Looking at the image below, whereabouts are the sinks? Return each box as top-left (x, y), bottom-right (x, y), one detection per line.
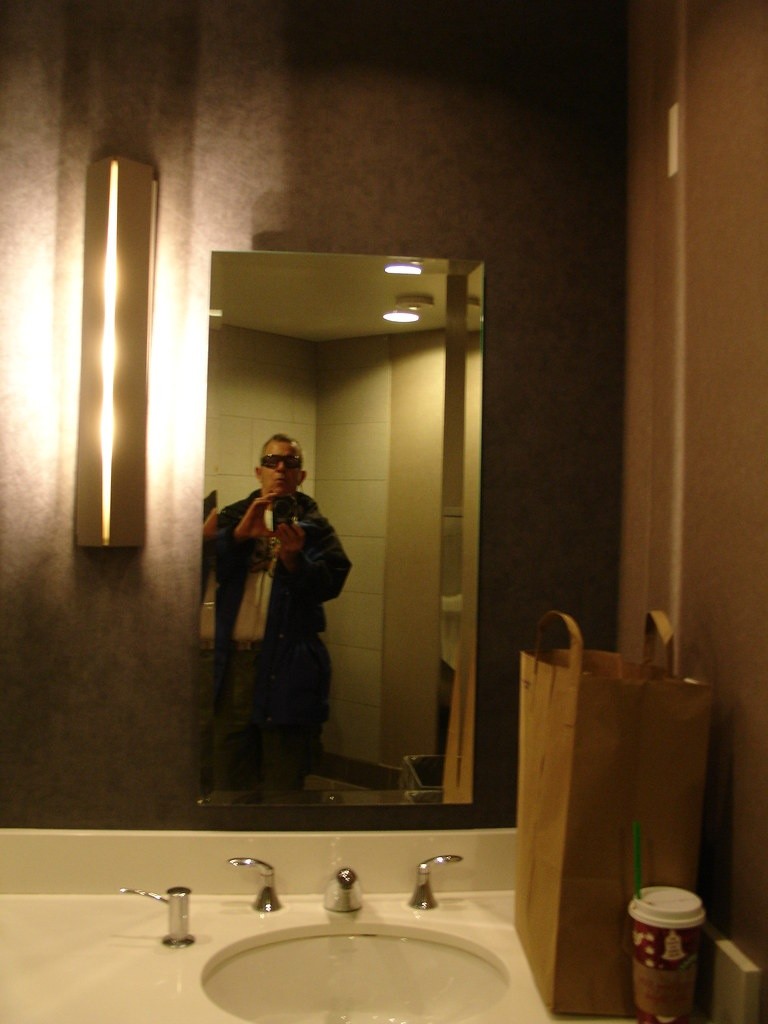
top-left (199, 920), bottom-right (510, 1024)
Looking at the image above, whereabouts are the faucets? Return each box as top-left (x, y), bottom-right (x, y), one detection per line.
top-left (323, 867), bottom-right (364, 911)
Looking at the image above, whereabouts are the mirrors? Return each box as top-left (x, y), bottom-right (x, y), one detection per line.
top-left (195, 249), bottom-right (484, 805)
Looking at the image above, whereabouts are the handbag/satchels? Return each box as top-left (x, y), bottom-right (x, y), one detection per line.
top-left (513, 609), bottom-right (711, 1017)
top-left (260, 634), bottom-right (331, 726)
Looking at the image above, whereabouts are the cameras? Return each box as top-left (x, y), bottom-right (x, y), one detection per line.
top-left (272, 495), bottom-right (297, 532)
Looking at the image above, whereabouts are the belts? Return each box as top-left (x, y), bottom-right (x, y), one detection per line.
top-left (233, 640), bottom-right (260, 651)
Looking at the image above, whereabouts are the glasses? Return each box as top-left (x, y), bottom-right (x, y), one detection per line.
top-left (262, 454), bottom-right (303, 468)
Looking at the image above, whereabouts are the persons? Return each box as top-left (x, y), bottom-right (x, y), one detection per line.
top-left (216, 433), bottom-right (353, 789)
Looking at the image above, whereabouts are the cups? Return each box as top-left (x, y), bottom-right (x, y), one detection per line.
top-left (630, 886), bottom-right (705, 1023)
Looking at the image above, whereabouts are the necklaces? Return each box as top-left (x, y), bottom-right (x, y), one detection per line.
top-left (269, 537), bottom-right (280, 576)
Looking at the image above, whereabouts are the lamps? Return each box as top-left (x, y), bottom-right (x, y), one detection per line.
top-left (72, 156), bottom-right (161, 550)
